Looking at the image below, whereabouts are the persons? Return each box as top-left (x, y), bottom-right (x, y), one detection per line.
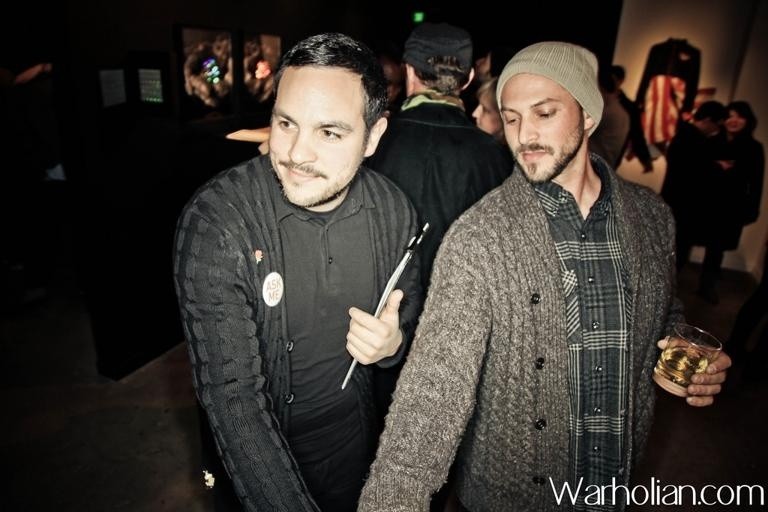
top-left (348, 38), bottom-right (736, 512)
top-left (170, 31), bottom-right (427, 512)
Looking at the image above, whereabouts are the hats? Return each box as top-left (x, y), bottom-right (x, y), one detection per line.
top-left (401, 21), bottom-right (472, 77)
top-left (496, 41), bottom-right (604, 137)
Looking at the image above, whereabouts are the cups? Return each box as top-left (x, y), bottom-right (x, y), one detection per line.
top-left (650, 323), bottom-right (723, 397)
